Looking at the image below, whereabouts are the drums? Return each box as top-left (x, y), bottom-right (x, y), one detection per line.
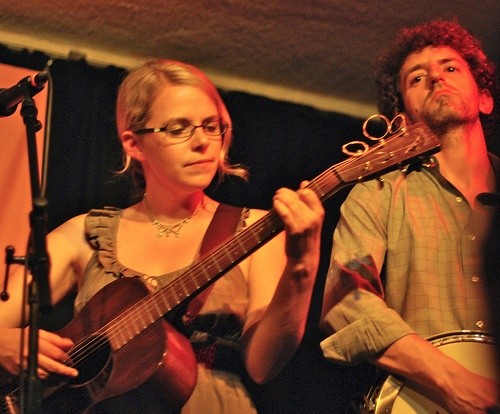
top-left (362, 330), bottom-right (500, 414)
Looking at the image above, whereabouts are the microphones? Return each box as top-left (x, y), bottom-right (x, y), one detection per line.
top-left (0, 72), bottom-right (48, 117)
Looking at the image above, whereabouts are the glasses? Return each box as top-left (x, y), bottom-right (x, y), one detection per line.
top-left (132, 118), bottom-right (230, 140)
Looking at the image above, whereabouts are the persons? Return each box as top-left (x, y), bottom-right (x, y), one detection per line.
top-left (318, 17), bottom-right (500, 414)
top-left (0, 58), bottom-right (326, 414)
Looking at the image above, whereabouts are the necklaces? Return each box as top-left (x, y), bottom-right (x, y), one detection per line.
top-left (143, 191), bottom-right (206, 238)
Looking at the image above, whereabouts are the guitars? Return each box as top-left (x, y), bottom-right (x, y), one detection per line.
top-left (0, 114), bottom-right (443, 414)
